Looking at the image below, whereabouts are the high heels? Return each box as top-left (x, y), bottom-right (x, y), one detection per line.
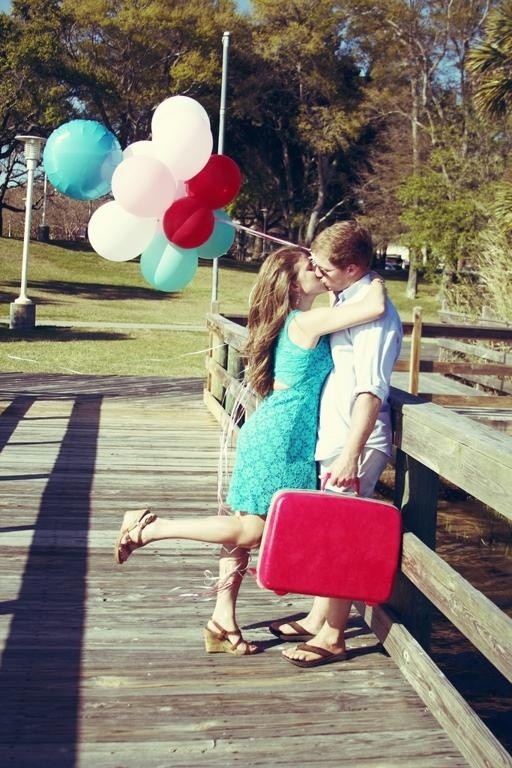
top-left (115, 509), bottom-right (158, 564)
top-left (203, 617), bottom-right (257, 655)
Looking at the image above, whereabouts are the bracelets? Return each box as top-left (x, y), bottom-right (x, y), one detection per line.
top-left (369, 278), bottom-right (385, 286)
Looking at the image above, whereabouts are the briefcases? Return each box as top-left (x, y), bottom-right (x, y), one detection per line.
top-left (256, 472), bottom-right (401, 606)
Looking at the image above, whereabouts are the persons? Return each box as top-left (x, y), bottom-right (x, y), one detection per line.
top-left (110, 245), bottom-right (389, 655)
top-left (264, 220), bottom-right (403, 667)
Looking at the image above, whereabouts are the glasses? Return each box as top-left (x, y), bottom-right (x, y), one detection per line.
top-left (316, 265), bottom-right (335, 276)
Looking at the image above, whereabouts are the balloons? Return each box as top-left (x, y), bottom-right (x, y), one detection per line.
top-left (45, 95), bottom-right (243, 292)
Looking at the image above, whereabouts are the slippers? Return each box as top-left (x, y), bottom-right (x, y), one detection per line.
top-left (269, 620), bottom-right (348, 668)
